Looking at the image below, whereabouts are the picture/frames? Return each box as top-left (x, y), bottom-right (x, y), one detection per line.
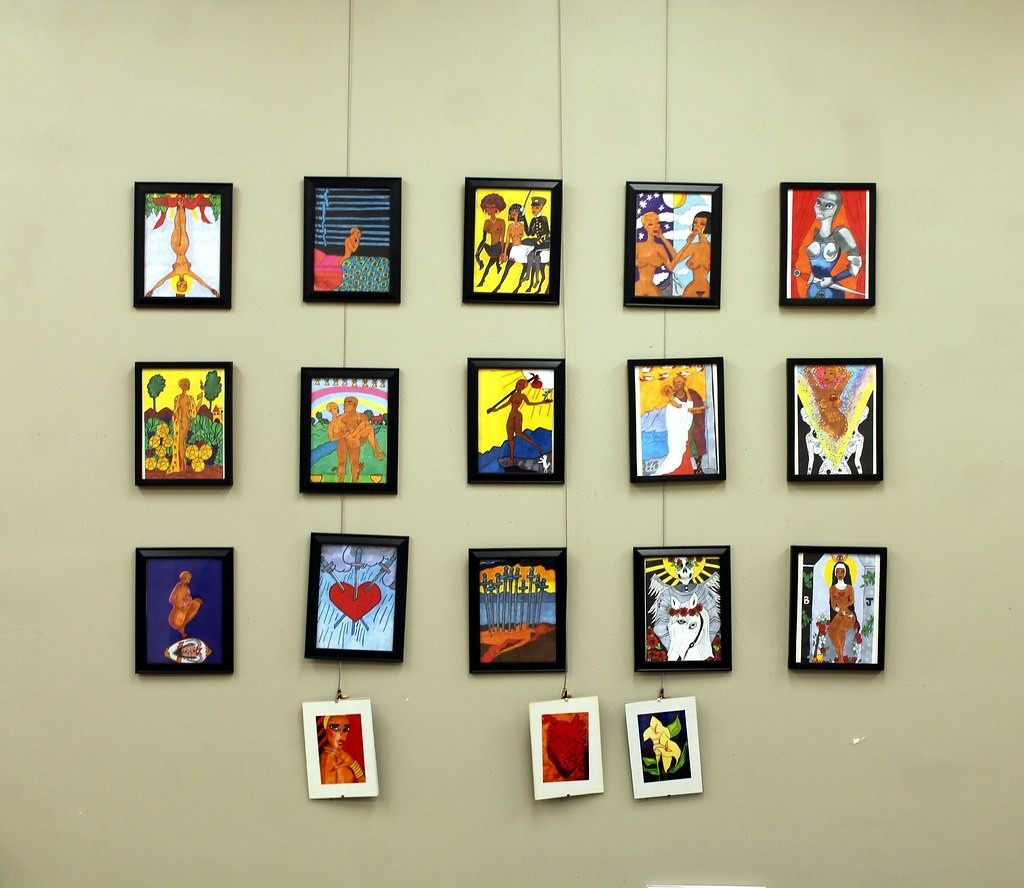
top-left (788, 544), bottom-right (887, 670)
top-left (134, 548), bottom-right (234, 675)
top-left (623, 180), bottom-right (722, 309)
top-left (467, 358), bottom-right (565, 484)
top-left (469, 547), bottom-right (568, 673)
top-left (134, 181), bottom-right (234, 309)
top-left (786, 358), bottom-right (883, 482)
top-left (305, 532), bottom-right (409, 663)
top-left (627, 357), bottom-right (726, 484)
top-left (463, 176), bottom-right (562, 305)
top-left (632, 545), bottom-right (733, 672)
top-left (779, 182), bottom-right (876, 307)
top-left (134, 362), bottom-right (234, 486)
top-left (303, 176), bottom-right (401, 304)
top-left (300, 367), bottom-right (399, 494)
